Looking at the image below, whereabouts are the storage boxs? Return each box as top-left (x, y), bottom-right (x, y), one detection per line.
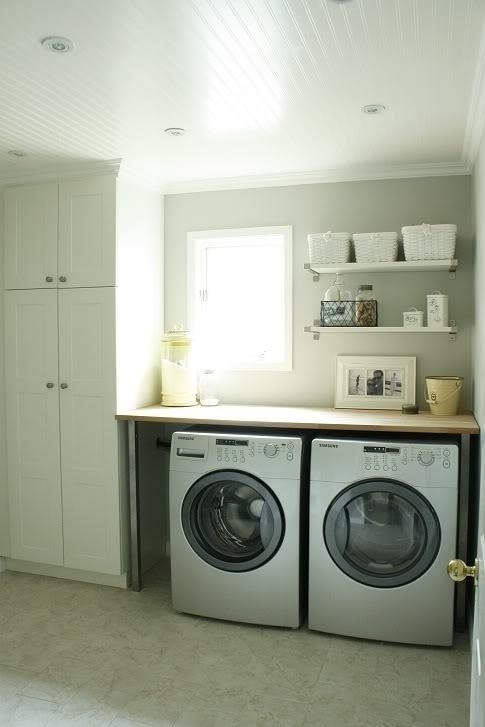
top-left (319, 300), bottom-right (378, 327)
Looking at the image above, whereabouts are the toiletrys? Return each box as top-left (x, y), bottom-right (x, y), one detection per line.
top-left (323, 273), bottom-right (353, 327)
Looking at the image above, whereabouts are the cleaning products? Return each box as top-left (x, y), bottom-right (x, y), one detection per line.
top-left (324, 271), bottom-right (356, 325)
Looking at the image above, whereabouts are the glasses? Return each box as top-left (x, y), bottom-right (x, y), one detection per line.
top-left (376, 376), bottom-right (381, 379)
top-left (367, 382), bottom-right (375, 387)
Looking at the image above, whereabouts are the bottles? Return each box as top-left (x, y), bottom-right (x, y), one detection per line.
top-left (355, 284), bottom-right (375, 326)
top-left (160, 322), bottom-right (198, 407)
top-left (198, 369), bottom-right (219, 406)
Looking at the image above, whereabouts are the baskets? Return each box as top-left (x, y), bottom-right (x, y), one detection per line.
top-left (308, 232), bottom-right (350, 264)
top-left (352, 231), bottom-right (398, 263)
top-left (401, 223), bottom-right (457, 261)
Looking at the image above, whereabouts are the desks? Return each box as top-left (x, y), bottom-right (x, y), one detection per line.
top-left (116, 399), bottom-right (479, 636)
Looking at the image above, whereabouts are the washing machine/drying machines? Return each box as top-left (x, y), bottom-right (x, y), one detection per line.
top-left (305, 438), bottom-right (459, 648)
top-left (167, 431), bottom-right (302, 632)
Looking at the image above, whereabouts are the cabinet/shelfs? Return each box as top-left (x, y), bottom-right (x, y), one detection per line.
top-left (0, 290), bottom-right (164, 592)
top-left (1, 159), bottom-right (166, 289)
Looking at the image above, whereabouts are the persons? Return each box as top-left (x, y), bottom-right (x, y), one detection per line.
top-left (389, 372), bottom-right (398, 396)
top-left (367, 370), bottom-right (383, 395)
top-left (356, 374), bottom-right (360, 389)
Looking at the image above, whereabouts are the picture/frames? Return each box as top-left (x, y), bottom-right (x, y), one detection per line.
top-left (335, 356), bottom-right (416, 412)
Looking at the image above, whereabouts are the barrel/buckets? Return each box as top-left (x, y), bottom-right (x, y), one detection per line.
top-left (423, 376), bottom-right (465, 416)
